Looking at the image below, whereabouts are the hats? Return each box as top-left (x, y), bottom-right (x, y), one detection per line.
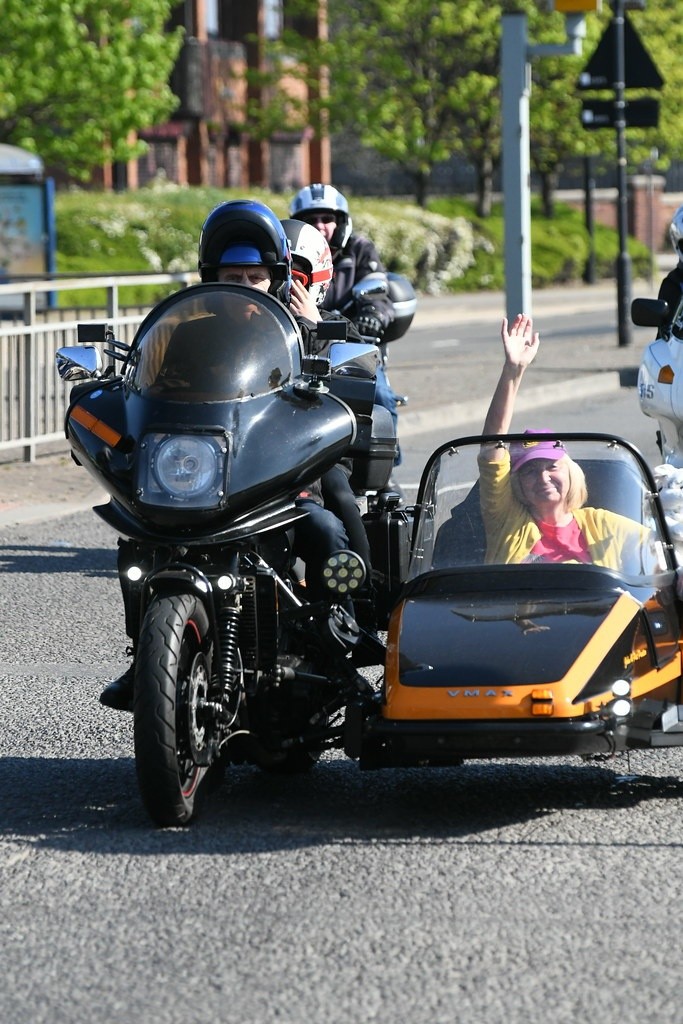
top-left (509, 430), bottom-right (565, 471)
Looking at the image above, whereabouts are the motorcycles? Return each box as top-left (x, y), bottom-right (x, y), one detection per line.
top-left (631, 284), bottom-right (682, 470)
top-left (52, 280), bottom-right (681, 828)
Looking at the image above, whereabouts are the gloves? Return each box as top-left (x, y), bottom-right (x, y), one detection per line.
top-left (355, 302), bottom-right (396, 339)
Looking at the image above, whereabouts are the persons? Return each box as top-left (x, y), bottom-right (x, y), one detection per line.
top-left (99, 200), bottom-right (372, 710)
top-left (277, 218), bottom-right (381, 668)
top-left (478, 314), bottom-right (667, 577)
top-left (654, 203), bottom-right (683, 343)
top-left (287, 184), bottom-right (405, 512)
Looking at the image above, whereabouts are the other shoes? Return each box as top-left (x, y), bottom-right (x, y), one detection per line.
top-left (100, 661), bottom-right (135, 712)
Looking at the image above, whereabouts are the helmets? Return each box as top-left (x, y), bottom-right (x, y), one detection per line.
top-left (669, 207), bottom-right (683, 262)
top-left (278, 218), bottom-right (333, 307)
top-left (198, 199), bottom-right (292, 308)
top-left (291, 183), bottom-right (354, 248)
